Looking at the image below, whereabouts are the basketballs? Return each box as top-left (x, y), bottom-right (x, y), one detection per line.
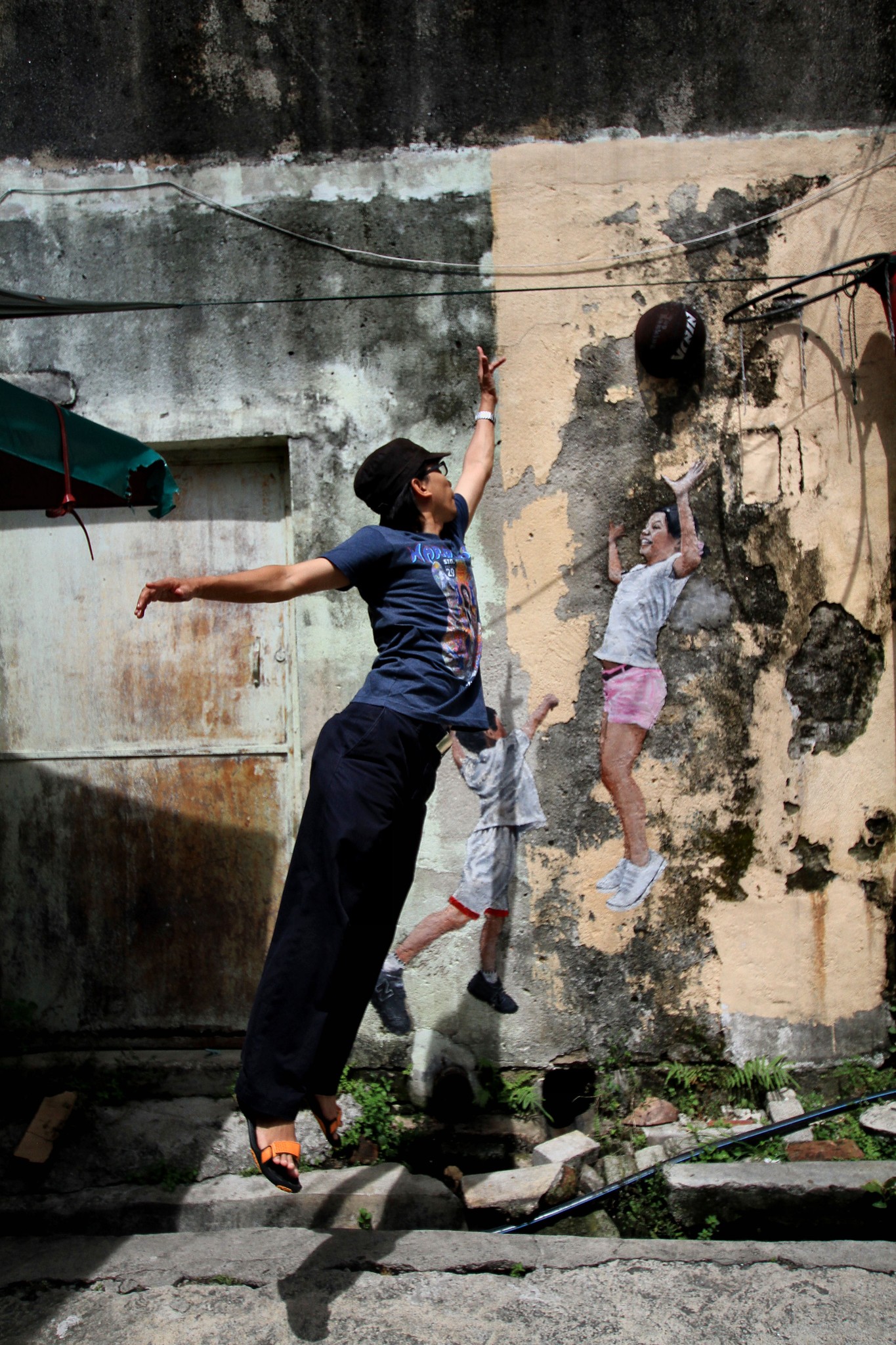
top-left (635, 301), bottom-right (705, 373)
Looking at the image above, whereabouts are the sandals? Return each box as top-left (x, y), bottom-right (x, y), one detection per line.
top-left (232, 1091), bottom-right (303, 1194)
top-left (307, 1093), bottom-right (342, 1149)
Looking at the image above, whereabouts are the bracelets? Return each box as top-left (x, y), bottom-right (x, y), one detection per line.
top-left (473, 410), bottom-right (497, 426)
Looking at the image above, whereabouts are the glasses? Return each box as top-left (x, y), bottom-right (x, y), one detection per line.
top-left (419, 461), bottom-right (448, 477)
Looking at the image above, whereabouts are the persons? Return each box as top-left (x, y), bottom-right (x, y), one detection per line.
top-left (131, 343), bottom-right (510, 1200)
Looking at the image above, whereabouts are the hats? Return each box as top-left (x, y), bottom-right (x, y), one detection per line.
top-left (353, 438), bottom-right (452, 516)
